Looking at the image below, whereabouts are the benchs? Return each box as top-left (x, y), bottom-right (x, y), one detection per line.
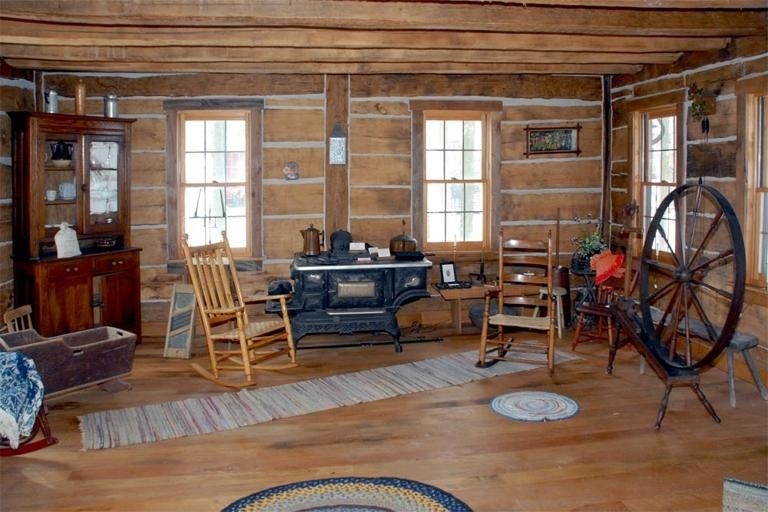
top-left (635, 299), bottom-right (767, 407)
top-left (608, 301), bottom-right (721, 430)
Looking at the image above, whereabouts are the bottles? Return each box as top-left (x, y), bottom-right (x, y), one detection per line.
top-left (106, 197), bottom-right (112, 223)
top-left (45, 88), bottom-right (57, 113)
top-left (74, 80), bottom-right (87, 115)
top-left (103, 85), bottom-right (120, 118)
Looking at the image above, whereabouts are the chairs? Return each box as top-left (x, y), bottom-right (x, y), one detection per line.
top-left (180, 232), bottom-right (298, 387)
top-left (0, 353), bottom-right (58, 458)
top-left (571, 266), bottom-right (639, 354)
top-left (2, 304), bottom-right (34, 330)
top-left (475, 227), bottom-right (561, 375)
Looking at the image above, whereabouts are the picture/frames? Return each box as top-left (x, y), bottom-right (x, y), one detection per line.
top-left (523, 124), bottom-right (582, 158)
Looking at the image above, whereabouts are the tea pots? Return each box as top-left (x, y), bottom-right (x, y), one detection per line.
top-left (300, 223), bottom-right (324, 256)
top-left (59, 176), bottom-right (76, 201)
top-left (51, 139), bottom-right (74, 160)
top-left (390, 218), bottom-right (417, 255)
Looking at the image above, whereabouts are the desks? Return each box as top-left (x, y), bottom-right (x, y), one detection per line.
top-left (570, 268), bottom-right (597, 329)
top-left (432, 282), bottom-right (491, 333)
top-left (267, 258), bottom-right (433, 355)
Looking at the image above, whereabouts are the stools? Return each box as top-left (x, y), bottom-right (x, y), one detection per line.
top-left (530, 286), bottom-right (566, 338)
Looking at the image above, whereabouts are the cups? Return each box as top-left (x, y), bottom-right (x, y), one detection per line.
top-left (46, 190), bottom-right (56, 201)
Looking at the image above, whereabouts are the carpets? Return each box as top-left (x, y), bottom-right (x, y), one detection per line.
top-left (78, 336), bottom-right (577, 452)
top-left (489, 390), bottom-right (580, 422)
top-left (218, 476), bottom-right (474, 512)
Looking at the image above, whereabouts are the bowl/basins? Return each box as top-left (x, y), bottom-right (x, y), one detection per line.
top-left (53, 160), bottom-right (71, 167)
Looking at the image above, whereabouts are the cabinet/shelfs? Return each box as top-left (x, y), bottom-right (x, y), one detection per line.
top-left (6, 110), bottom-right (141, 346)
